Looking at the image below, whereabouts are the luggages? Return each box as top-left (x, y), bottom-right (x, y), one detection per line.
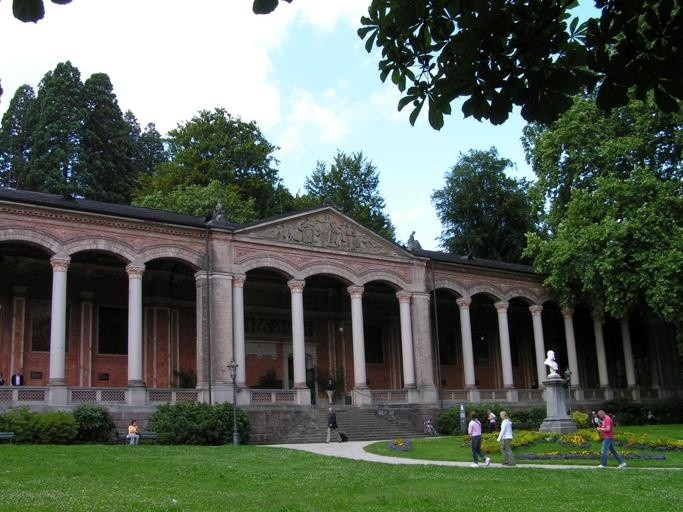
top-left (338, 432), bottom-right (348, 443)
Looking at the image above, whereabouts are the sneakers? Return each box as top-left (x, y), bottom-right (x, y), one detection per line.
top-left (485, 457), bottom-right (491, 467)
top-left (617, 463), bottom-right (626, 469)
top-left (503, 461), bottom-right (516, 466)
top-left (597, 464), bottom-right (607, 468)
top-left (470, 464), bottom-right (479, 468)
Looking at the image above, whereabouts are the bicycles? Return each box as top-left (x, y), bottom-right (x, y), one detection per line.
top-left (422, 415), bottom-right (438, 437)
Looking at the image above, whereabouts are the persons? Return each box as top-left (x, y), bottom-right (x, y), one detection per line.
top-left (10, 370), bottom-right (23, 385)
top-left (543, 349), bottom-right (558, 371)
top-left (596, 409), bottom-right (627, 468)
top-left (495, 411), bottom-right (514, 466)
top-left (467, 410), bottom-right (490, 468)
top-left (126, 420), bottom-right (139, 446)
top-left (487, 409), bottom-right (498, 433)
top-left (324, 377), bottom-right (335, 404)
top-left (325, 407), bottom-right (342, 442)
top-left (0, 372), bottom-right (3, 385)
top-left (591, 410), bottom-right (616, 427)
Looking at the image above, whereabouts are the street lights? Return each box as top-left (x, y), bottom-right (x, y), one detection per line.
top-left (225, 357), bottom-right (242, 445)
top-left (563, 365), bottom-right (573, 416)
